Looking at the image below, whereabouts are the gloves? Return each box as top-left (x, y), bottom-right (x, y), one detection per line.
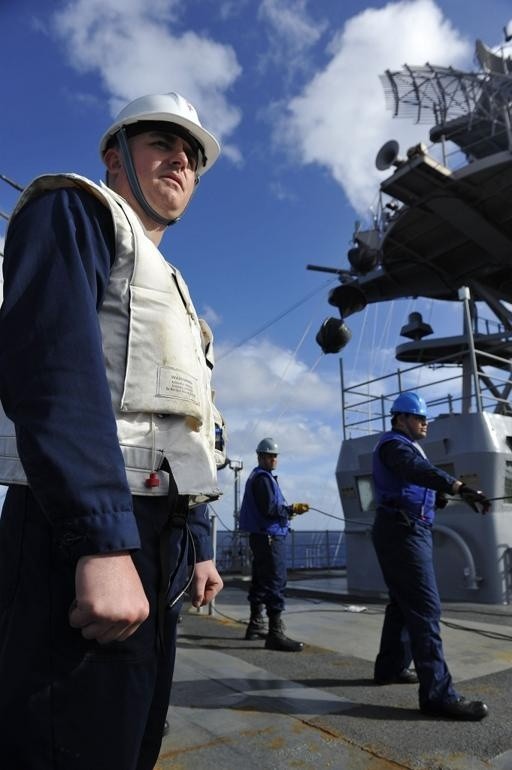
top-left (458, 483), bottom-right (491, 515)
top-left (292, 503), bottom-right (310, 515)
top-left (436, 491), bottom-right (447, 509)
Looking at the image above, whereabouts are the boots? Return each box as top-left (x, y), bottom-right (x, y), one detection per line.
top-left (245, 602), bottom-right (270, 641)
top-left (265, 611), bottom-right (304, 652)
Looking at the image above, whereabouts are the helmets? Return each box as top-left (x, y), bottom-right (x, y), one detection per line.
top-left (255, 438), bottom-right (280, 454)
top-left (390, 391), bottom-right (427, 417)
top-left (99, 92), bottom-right (221, 183)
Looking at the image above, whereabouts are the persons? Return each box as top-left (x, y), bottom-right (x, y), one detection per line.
top-left (236, 437), bottom-right (311, 653)
top-left (0, 87), bottom-right (227, 770)
top-left (369, 390), bottom-right (492, 723)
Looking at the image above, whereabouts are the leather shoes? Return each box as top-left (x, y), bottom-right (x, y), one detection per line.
top-left (373, 668), bottom-right (419, 685)
top-left (422, 695), bottom-right (487, 721)
